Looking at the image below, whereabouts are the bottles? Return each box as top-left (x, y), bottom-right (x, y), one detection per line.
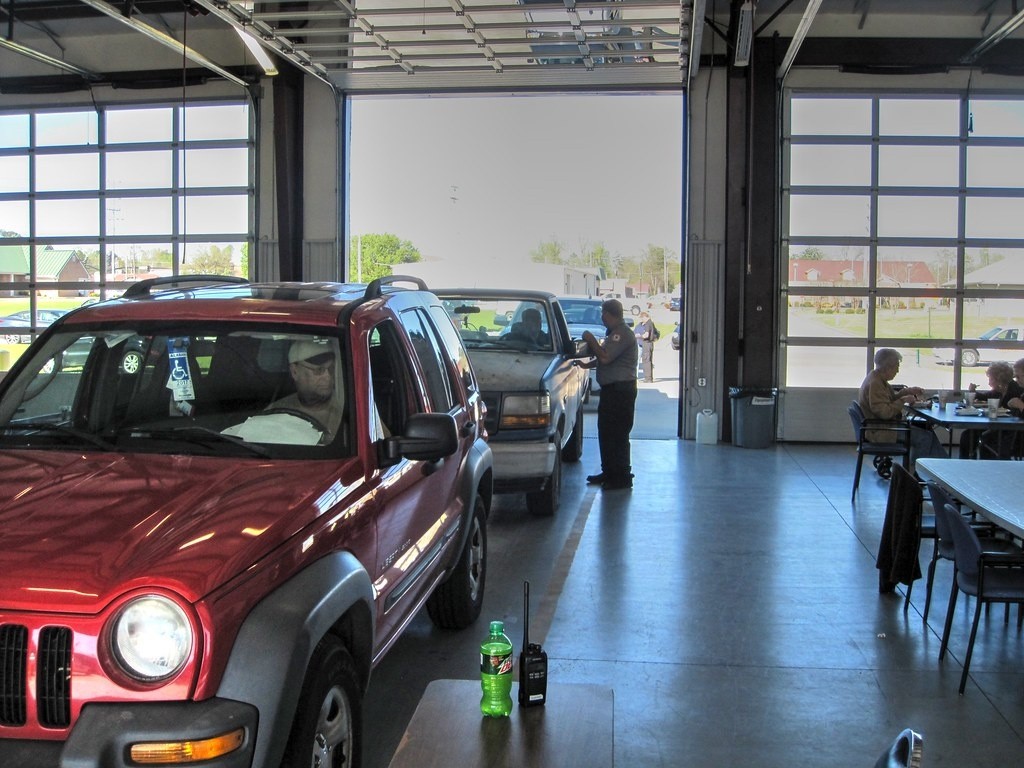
top-left (480, 621), bottom-right (513, 718)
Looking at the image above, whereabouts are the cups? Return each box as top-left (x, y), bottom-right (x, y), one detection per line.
top-left (988, 399), bottom-right (1000, 418)
top-left (965, 393), bottom-right (976, 408)
top-left (938, 391), bottom-right (949, 408)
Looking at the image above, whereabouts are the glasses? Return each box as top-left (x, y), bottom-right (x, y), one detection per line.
top-left (295, 363), bottom-right (335, 376)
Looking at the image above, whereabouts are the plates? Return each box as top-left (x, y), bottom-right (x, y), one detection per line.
top-left (973, 401), bottom-right (988, 408)
top-left (909, 402), bottom-right (933, 408)
top-left (986, 408), bottom-right (1011, 414)
top-left (953, 409), bottom-right (983, 415)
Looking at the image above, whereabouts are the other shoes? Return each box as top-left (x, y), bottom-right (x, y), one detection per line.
top-left (586, 470), bottom-right (606, 483)
top-left (601, 471), bottom-right (633, 490)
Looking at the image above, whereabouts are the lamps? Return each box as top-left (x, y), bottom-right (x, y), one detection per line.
top-left (233, 25), bottom-right (278, 75)
top-left (733, 0), bottom-right (756, 67)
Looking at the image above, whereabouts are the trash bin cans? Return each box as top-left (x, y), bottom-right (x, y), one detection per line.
top-left (729, 386), bottom-right (777, 449)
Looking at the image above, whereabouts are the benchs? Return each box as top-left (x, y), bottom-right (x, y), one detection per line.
top-left (180, 338), bottom-right (397, 419)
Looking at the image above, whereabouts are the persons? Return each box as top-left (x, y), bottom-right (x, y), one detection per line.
top-left (576, 299), bottom-right (638, 490)
top-left (507, 308), bottom-right (551, 347)
top-left (587, 309), bottom-right (602, 324)
top-left (859, 349), bottom-right (948, 485)
top-left (265, 340), bottom-right (390, 442)
top-left (633, 312), bottom-right (654, 383)
top-left (959, 358), bottom-right (1024, 461)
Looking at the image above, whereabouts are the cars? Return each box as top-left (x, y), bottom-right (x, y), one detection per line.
top-left (0, 309), bottom-right (162, 377)
top-left (672, 321), bottom-right (681, 350)
top-left (494, 297), bottom-right (634, 396)
top-left (669, 298), bottom-right (681, 311)
top-left (933, 324), bottom-right (1024, 368)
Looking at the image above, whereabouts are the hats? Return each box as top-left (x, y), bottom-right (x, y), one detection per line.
top-left (287, 339), bottom-right (336, 364)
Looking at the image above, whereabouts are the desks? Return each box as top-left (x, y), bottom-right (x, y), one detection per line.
top-left (389, 679), bottom-right (615, 768)
top-left (916, 457), bottom-right (1024, 624)
top-left (903, 401), bottom-right (1024, 527)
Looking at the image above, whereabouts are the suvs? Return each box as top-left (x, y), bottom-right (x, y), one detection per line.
top-left (0, 274), bottom-right (496, 768)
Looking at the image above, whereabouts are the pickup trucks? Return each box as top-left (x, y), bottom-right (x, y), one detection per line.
top-left (428, 288), bottom-right (591, 519)
top-left (598, 290), bottom-right (649, 317)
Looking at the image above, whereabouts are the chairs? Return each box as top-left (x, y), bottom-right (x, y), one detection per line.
top-left (111, 353), bottom-right (208, 430)
top-left (847, 400), bottom-right (1024, 695)
top-left (510, 320), bottom-right (524, 335)
top-left (275, 350), bottom-right (393, 430)
